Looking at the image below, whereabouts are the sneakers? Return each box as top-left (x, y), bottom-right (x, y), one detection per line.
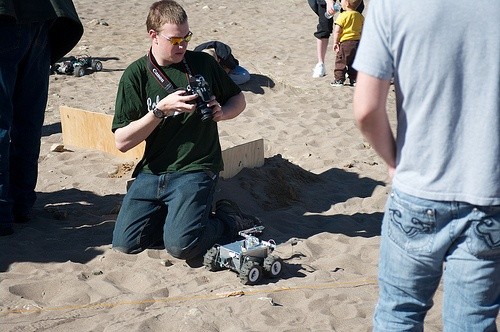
top-left (215, 198), bottom-right (260, 230)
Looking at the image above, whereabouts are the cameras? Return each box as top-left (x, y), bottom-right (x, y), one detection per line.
top-left (185, 78), bottom-right (214, 122)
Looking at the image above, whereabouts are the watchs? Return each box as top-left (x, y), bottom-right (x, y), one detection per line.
top-left (152, 106), bottom-right (166, 123)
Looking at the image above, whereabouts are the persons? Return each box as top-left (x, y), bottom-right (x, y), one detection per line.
top-left (111, 0), bottom-right (263, 261)
top-left (308, 0), bottom-right (365, 78)
top-left (331, 0), bottom-right (365, 88)
top-left (352, 0), bottom-right (500, 332)
top-left (0, 0), bottom-right (83, 232)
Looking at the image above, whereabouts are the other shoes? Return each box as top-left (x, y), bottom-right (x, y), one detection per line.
top-left (331, 80), bottom-right (343, 87)
top-left (313, 63), bottom-right (326, 79)
top-left (0, 205), bottom-right (15, 235)
top-left (13, 191), bottom-right (37, 224)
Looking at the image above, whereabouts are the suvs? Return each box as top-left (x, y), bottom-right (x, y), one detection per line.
top-left (202, 225), bottom-right (284, 286)
top-left (49, 56), bottom-right (102, 77)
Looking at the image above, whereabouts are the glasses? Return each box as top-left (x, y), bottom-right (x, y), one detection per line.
top-left (158, 30), bottom-right (193, 46)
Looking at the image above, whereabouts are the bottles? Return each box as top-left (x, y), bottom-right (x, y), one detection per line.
top-left (324, 3), bottom-right (341, 19)
